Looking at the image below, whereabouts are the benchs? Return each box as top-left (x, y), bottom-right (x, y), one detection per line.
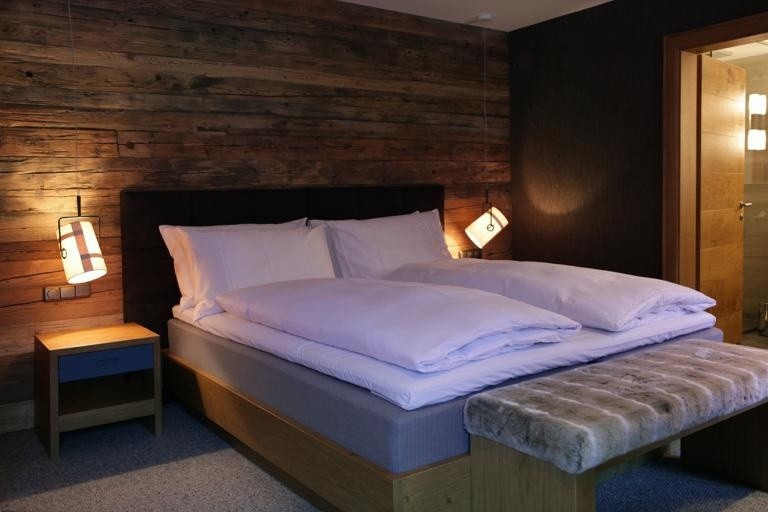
top-left (462, 336), bottom-right (768, 512)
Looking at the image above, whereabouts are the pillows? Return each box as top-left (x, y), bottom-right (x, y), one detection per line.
top-left (155, 216), bottom-right (309, 314)
top-left (304, 211), bottom-right (421, 279)
top-left (321, 209), bottom-right (454, 281)
top-left (169, 223), bottom-right (340, 328)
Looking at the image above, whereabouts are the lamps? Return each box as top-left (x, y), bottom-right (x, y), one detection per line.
top-left (462, 9), bottom-right (511, 253)
top-left (50, 1), bottom-right (108, 289)
top-left (745, 91), bottom-right (768, 151)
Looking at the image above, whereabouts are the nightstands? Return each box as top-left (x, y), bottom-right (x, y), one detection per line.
top-left (34, 321), bottom-right (164, 463)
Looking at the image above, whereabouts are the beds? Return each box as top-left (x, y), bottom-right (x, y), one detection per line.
top-left (113, 178), bottom-right (725, 512)
